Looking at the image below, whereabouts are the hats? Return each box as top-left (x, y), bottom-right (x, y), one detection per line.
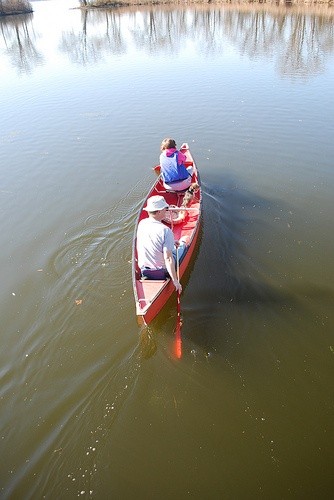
top-left (143, 195), bottom-right (169, 211)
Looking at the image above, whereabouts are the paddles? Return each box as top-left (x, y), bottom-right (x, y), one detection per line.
top-left (174, 242), bottom-right (182, 360)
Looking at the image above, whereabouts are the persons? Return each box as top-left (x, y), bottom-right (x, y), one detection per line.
top-left (160, 138), bottom-right (193, 191)
top-left (136, 195), bottom-right (189, 295)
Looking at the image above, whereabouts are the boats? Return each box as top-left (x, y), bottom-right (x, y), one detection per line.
top-left (131, 143), bottom-right (202, 330)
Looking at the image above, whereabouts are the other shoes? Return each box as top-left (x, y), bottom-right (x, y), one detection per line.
top-left (179, 236), bottom-right (191, 245)
